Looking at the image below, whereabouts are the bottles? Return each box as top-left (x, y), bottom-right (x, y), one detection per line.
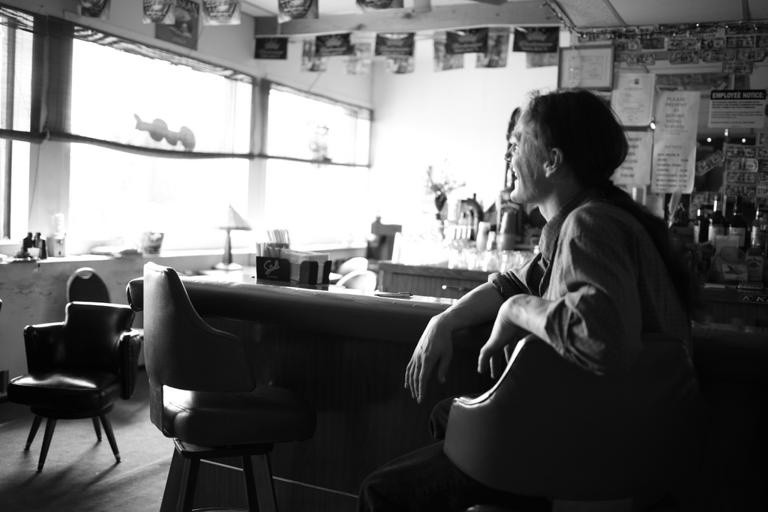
top-left (708, 195), bottom-right (725, 243)
top-left (750, 205), bottom-right (764, 252)
top-left (693, 208), bottom-right (707, 244)
top-left (729, 195), bottom-right (747, 251)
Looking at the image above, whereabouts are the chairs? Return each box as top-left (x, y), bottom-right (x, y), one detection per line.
top-left (442, 333), bottom-right (695, 512)
top-left (7, 300), bottom-right (143, 474)
top-left (66, 267), bottom-right (144, 339)
top-left (144, 260), bottom-right (316, 512)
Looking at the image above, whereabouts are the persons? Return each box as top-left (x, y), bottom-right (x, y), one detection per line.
top-left (355, 88), bottom-right (703, 512)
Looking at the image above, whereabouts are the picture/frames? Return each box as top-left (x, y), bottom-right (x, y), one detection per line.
top-left (557, 44), bottom-right (615, 92)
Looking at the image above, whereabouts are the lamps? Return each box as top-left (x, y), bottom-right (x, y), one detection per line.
top-left (211, 204), bottom-right (251, 270)
